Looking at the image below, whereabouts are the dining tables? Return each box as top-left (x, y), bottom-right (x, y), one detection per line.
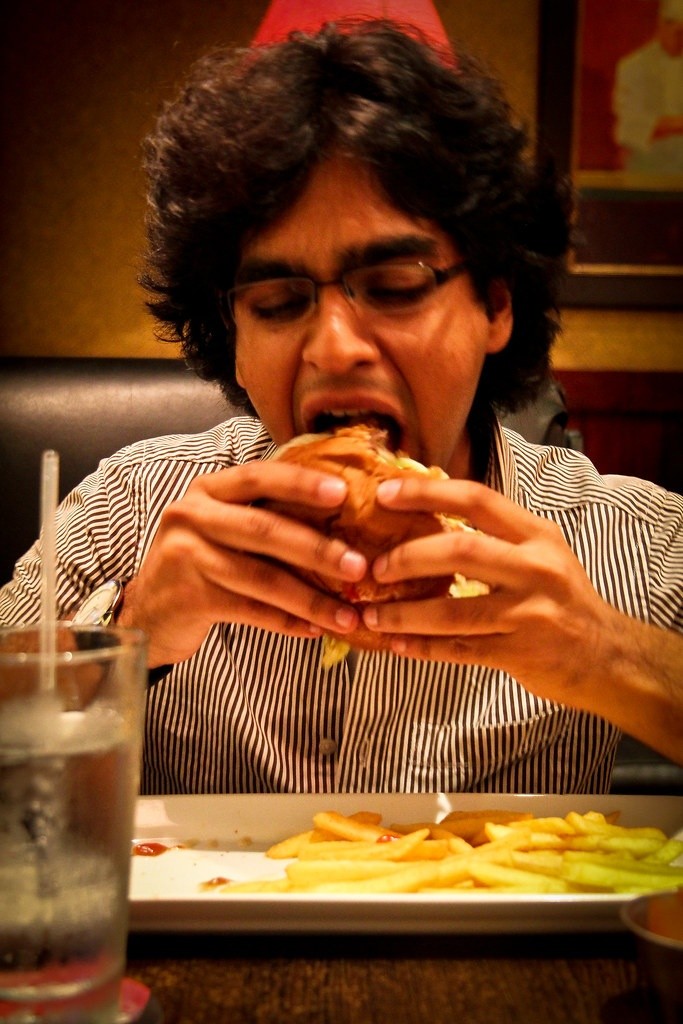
top-left (124, 930), bottom-right (683, 1024)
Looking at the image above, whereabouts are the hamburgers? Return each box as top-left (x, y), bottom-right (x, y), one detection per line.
top-left (261, 422), bottom-right (498, 671)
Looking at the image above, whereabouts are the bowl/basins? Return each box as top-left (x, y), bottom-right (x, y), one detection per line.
top-left (619, 889), bottom-right (682, 1010)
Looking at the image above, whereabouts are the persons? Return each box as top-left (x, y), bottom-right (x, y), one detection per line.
top-left (0, 14), bottom-right (683, 795)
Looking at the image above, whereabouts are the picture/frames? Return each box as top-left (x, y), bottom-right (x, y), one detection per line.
top-left (531, 0), bottom-right (683, 315)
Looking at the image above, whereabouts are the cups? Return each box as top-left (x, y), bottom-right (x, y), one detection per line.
top-left (1, 618), bottom-right (148, 1021)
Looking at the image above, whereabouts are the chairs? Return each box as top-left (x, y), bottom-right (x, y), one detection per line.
top-left (0, 356), bottom-right (248, 591)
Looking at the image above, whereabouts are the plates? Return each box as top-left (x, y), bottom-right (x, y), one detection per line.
top-left (103, 788), bottom-right (682, 936)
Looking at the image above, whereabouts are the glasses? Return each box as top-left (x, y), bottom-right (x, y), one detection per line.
top-left (222, 247), bottom-right (489, 334)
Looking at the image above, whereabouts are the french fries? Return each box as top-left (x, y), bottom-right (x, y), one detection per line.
top-left (222, 811), bottom-right (683, 896)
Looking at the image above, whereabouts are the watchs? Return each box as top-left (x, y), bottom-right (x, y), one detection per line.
top-left (67, 573), bottom-right (174, 693)
